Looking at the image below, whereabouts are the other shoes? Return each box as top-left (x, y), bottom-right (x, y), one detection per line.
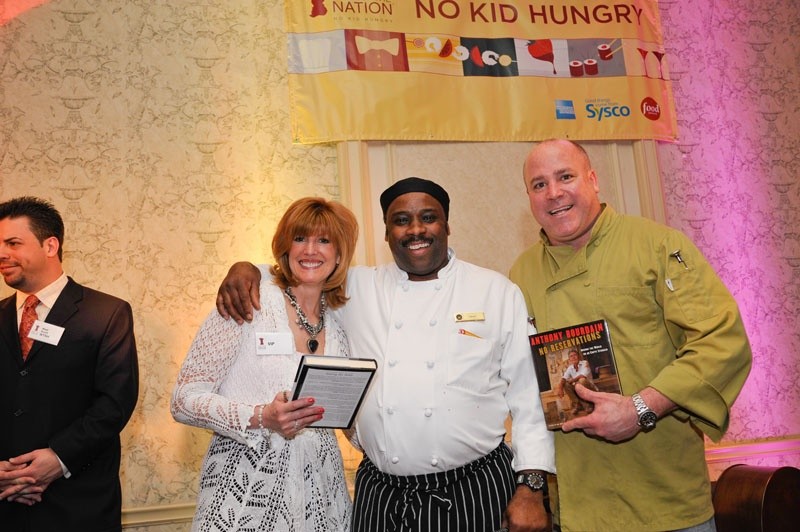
top-left (585, 402), bottom-right (595, 413)
top-left (572, 403), bottom-right (583, 415)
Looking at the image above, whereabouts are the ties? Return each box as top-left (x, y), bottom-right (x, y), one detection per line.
top-left (18, 293), bottom-right (39, 361)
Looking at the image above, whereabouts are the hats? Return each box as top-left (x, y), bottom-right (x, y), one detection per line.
top-left (380, 176), bottom-right (449, 220)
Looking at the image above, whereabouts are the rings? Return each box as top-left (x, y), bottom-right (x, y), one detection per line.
top-left (294, 420), bottom-right (302, 429)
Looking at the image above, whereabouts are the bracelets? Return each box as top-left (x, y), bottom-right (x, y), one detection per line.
top-left (257, 404), bottom-right (274, 450)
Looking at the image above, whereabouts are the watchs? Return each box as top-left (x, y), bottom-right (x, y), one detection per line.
top-left (515, 474), bottom-right (548, 491)
top-left (632, 393), bottom-right (658, 431)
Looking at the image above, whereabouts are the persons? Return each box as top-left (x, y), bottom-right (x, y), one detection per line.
top-left (1, 195), bottom-right (141, 532)
top-left (553, 348), bottom-right (599, 421)
top-left (172, 202), bottom-right (367, 532)
top-left (217, 176), bottom-right (560, 532)
top-left (501, 137), bottom-right (753, 532)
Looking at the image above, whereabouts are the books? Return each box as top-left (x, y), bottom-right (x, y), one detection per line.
top-left (288, 355), bottom-right (378, 430)
top-left (524, 320), bottom-right (624, 432)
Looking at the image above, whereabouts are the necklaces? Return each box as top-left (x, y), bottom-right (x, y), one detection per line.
top-left (284, 285), bottom-right (329, 354)
